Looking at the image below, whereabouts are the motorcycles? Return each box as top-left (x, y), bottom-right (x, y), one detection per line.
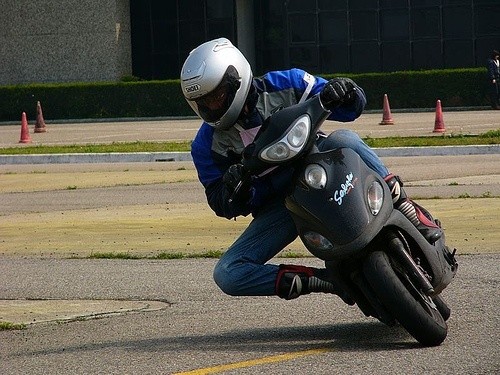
top-left (201, 83), bottom-right (458, 346)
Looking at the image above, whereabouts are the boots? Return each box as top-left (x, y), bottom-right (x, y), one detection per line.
top-left (383, 172), bottom-right (442, 245)
top-left (275, 264), bottom-right (355, 305)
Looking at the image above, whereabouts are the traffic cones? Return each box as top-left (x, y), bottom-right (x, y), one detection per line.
top-left (433, 100), bottom-right (446, 132)
top-left (33, 100), bottom-right (47, 133)
top-left (379, 94), bottom-right (394, 125)
top-left (18, 112), bottom-right (32, 143)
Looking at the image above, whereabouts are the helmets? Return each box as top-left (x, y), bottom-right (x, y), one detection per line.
top-left (180, 38), bottom-right (252, 130)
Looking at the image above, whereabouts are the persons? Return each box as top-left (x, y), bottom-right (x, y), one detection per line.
top-left (487, 50), bottom-right (500, 109)
top-left (180, 36), bottom-right (447, 307)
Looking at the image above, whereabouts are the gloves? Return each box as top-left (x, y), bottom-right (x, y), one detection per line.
top-left (324, 77), bottom-right (356, 106)
top-left (220, 165), bottom-right (255, 202)
